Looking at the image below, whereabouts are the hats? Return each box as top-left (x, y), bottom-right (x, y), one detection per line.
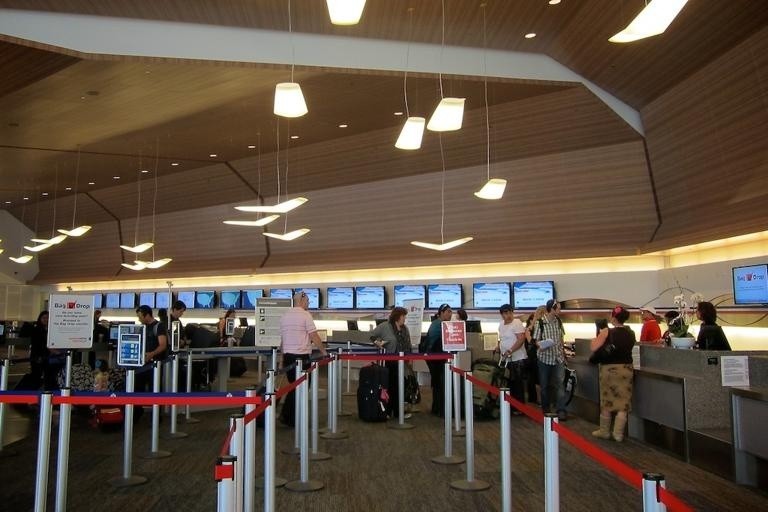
top-left (639, 307), bottom-right (656, 315)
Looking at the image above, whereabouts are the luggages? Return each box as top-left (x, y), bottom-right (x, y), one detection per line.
top-left (356, 346), bottom-right (392, 422)
top-left (472, 349), bottom-right (511, 416)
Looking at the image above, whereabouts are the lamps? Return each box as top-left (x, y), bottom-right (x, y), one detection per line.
top-left (410, 133), bottom-right (474, 251)
top-left (525, 33), bottom-right (536, 38)
top-left (210, 154), bottom-right (217, 157)
top-left (327, 1), bottom-right (365, 25)
top-left (222, 114), bottom-right (311, 240)
top-left (274, 1), bottom-right (308, 118)
top-left (340, 124), bottom-right (347, 127)
top-left (88, 182), bottom-right (95, 186)
top-left (394, 111), bottom-right (403, 115)
top-left (395, 8), bottom-right (425, 150)
top-left (8, 146), bottom-right (92, 264)
top-left (474, 4), bottom-right (507, 200)
top-left (114, 177), bottom-right (121, 179)
top-left (172, 163), bottom-right (179, 166)
top-left (426, 1), bottom-right (465, 133)
top-left (608, 1), bottom-right (688, 43)
top-left (549, 1), bottom-right (560, 5)
top-left (120, 138), bottom-right (172, 271)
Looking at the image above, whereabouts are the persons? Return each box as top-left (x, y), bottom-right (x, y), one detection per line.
top-left (661, 311), bottom-right (694, 348)
top-left (423, 303), bottom-right (452, 417)
top-left (279, 291), bottom-right (327, 426)
top-left (136, 301), bottom-right (191, 394)
top-left (217, 310), bottom-right (237, 347)
top-left (494, 299), bottom-right (568, 422)
top-left (369, 307), bottom-right (413, 420)
top-left (591, 306), bottom-right (636, 443)
top-left (30, 311), bottom-right (53, 386)
top-left (94, 310), bottom-right (104, 342)
top-left (639, 304), bottom-right (663, 343)
top-left (689, 303), bottom-right (731, 349)
top-left (88, 360), bottom-right (109, 410)
top-left (456, 308), bottom-right (468, 322)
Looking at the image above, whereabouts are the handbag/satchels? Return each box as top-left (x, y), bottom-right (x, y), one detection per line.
top-left (405, 364), bottom-right (421, 405)
top-left (588, 327), bottom-right (615, 365)
top-left (359, 364), bottom-right (390, 388)
top-left (564, 364), bottom-right (577, 392)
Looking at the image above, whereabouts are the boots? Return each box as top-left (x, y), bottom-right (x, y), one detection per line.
top-left (591, 414), bottom-right (612, 440)
top-left (610, 416), bottom-right (628, 442)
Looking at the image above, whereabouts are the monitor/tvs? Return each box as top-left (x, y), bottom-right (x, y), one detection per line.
top-left (427, 284), bottom-right (462, 309)
top-left (94, 289), bottom-right (293, 310)
top-left (355, 286), bottom-right (385, 309)
top-left (732, 263), bottom-right (768, 304)
top-left (472, 282), bottom-right (511, 309)
top-left (513, 281), bottom-right (554, 310)
top-left (326, 287), bottom-right (354, 309)
top-left (294, 288), bottom-right (320, 309)
top-left (394, 285), bottom-right (426, 309)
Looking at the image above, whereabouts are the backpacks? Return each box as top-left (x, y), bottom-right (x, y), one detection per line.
top-left (153, 321), bottom-right (172, 362)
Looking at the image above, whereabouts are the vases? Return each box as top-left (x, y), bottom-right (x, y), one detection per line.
top-left (669, 337), bottom-right (698, 351)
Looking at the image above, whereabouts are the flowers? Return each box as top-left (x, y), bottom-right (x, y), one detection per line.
top-left (662, 288), bottom-right (705, 337)
